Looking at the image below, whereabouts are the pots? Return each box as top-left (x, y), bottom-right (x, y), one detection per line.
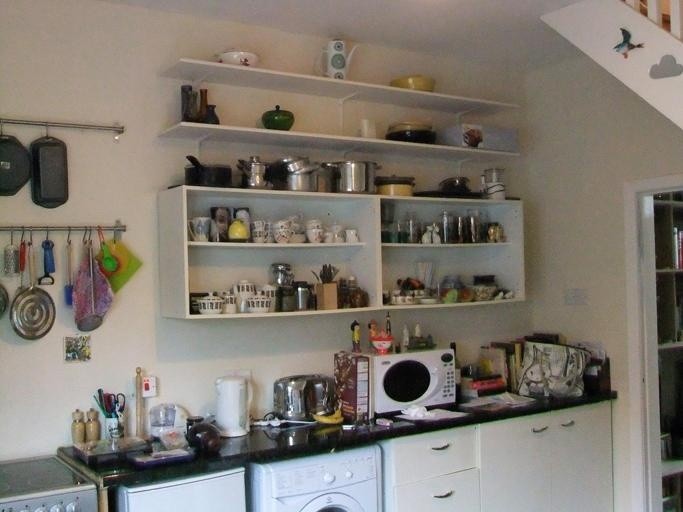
top-left (184, 154), bottom-right (381, 192)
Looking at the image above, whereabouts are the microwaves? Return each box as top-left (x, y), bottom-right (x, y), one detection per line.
top-left (373, 347), bottom-right (456, 414)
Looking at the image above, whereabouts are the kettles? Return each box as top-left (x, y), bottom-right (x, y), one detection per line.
top-left (213, 376), bottom-right (253, 438)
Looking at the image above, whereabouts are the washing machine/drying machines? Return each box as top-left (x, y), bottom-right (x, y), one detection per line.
top-left (245, 443), bottom-right (383, 512)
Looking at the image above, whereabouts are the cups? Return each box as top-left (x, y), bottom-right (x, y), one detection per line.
top-left (188, 211), bottom-right (360, 244)
top-left (106, 417), bottom-right (123, 440)
top-left (262, 285), bottom-right (276, 310)
top-left (220, 292), bottom-right (237, 313)
top-left (484, 167), bottom-right (507, 200)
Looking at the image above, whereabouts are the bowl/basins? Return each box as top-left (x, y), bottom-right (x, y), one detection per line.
top-left (213, 50), bottom-right (259, 66)
top-left (469, 285), bottom-right (497, 301)
top-left (246, 300), bottom-right (271, 314)
top-left (385, 122), bottom-right (436, 143)
top-left (389, 72), bottom-right (438, 91)
top-left (197, 300), bottom-right (223, 314)
top-left (370, 337), bottom-right (393, 354)
top-left (376, 176), bottom-right (415, 197)
top-left (184, 424), bottom-right (222, 456)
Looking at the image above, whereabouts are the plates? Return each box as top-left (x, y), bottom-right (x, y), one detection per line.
top-left (415, 297), bottom-right (440, 304)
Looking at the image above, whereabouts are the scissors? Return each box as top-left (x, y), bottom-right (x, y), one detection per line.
top-left (109, 393), bottom-right (125, 413)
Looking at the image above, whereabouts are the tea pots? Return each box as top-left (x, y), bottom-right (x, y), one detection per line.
top-left (232, 279), bottom-right (255, 312)
top-left (317, 37), bottom-right (357, 81)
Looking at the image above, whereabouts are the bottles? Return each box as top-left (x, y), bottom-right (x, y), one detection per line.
top-left (461, 345), bottom-right (492, 400)
top-left (271, 261), bottom-right (310, 310)
top-left (401, 210), bottom-right (419, 242)
top-left (187, 416), bottom-right (203, 433)
top-left (439, 210), bottom-right (482, 244)
top-left (182, 85), bottom-right (218, 124)
top-left (71, 409), bottom-right (101, 442)
top-left (441, 282), bottom-right (454, 304)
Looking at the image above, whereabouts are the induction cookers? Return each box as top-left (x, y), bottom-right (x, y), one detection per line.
top-left (0, 456), bottom-right (98, 512)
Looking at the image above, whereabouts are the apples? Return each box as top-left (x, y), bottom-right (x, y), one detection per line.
top-left (262, 105), bottom-right (294, 130)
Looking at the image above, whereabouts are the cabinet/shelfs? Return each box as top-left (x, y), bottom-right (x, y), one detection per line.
top-left (382, 401), bottom-right (614, 512)
top-left (654, 191), bottom-right (683, 512)
top-left (160, 118), bottom-right (525, 165)
top-left (161, 58), bottom-right (526, 118)
top-left (158, 186), bottom-right (524, 319)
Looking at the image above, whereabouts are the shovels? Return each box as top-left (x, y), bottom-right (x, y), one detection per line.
top-left (101, 242), bottom-right (118, 273)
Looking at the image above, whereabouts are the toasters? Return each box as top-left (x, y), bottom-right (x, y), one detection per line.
top-left (273, 375), bottom-right (337, 422)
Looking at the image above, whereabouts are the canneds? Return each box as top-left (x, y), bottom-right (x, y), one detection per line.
top-left (210, 206), bottom-right (230, 241)
top-left (233, 207), bottom-right (251, 241)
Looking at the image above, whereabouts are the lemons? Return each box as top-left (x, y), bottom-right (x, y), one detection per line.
top-left (228, 220), bottom-right (246, 239)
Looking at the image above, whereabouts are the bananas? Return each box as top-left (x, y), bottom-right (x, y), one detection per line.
top-left (309, 409), bottom-right (344, 424)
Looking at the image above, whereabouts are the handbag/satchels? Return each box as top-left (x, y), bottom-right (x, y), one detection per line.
top-left (513, 340), bottom-right (592, 399)
